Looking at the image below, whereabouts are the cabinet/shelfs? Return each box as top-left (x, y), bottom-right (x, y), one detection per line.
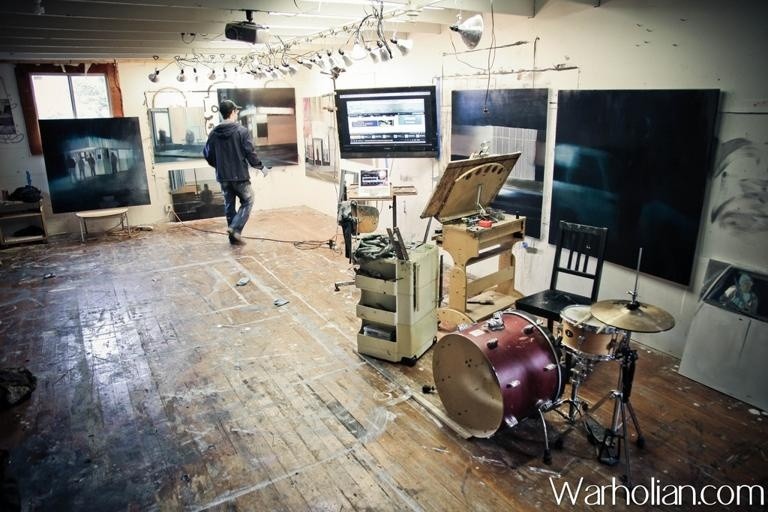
top-left (0, 198), bottom-right (48, 249)
top-left (355, 246), bottom-right (438, 367)
top-left (437, 213), bottom-right (527, 331)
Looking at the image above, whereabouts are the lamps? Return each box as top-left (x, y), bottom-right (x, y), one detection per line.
top-left (148, 55), bottom-right (160, 83)
top-left (359, 17), bottom-right (414, 62)
top-left (175, 54), bottom-right (216, 82)
top-left (449, 0), bottom-right (485, 49)
top-left (246, 28), bottom-right (352, 79)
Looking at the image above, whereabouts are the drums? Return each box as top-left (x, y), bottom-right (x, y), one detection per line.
top-left (431, 309), bottom-right (565, 442)
top-left (559, 305), bottom-right (626, 360)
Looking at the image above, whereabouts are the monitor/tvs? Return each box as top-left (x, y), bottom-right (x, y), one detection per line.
top-left (333, 85), bottom-right (441, 159)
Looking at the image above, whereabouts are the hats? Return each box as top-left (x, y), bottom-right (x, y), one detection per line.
top-left (220, 99), bottom-right (242, 117)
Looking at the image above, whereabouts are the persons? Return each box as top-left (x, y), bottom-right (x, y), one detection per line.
top-left (87, 153), bottom-right (95, 176)
top-left (110, 152), bottom-right (120, 176)
top-left (65, 155), bottom-right (78, 184)
top-left (77, 156), bottom-right (86, 182)
top-left (202, 100), bottom-right (269, 245)
top-left (718, 272), bottom-right (759, 313)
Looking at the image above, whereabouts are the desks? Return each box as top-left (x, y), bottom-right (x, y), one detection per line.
top-left (75, 206), bottom-right (130, 243)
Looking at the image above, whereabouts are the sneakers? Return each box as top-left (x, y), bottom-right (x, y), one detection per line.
top-left (226, 226), bottom-right (248, 246)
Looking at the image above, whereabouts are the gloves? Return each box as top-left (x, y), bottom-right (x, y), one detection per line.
top-left (261, 166), bottom-right (269, 178)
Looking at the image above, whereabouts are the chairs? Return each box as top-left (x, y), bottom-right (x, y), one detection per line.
top-left (516, 221), bottom-right (608, 332)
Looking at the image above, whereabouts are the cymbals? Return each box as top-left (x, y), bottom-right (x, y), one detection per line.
top-left (592, 300), bottom-right (674, 334)
